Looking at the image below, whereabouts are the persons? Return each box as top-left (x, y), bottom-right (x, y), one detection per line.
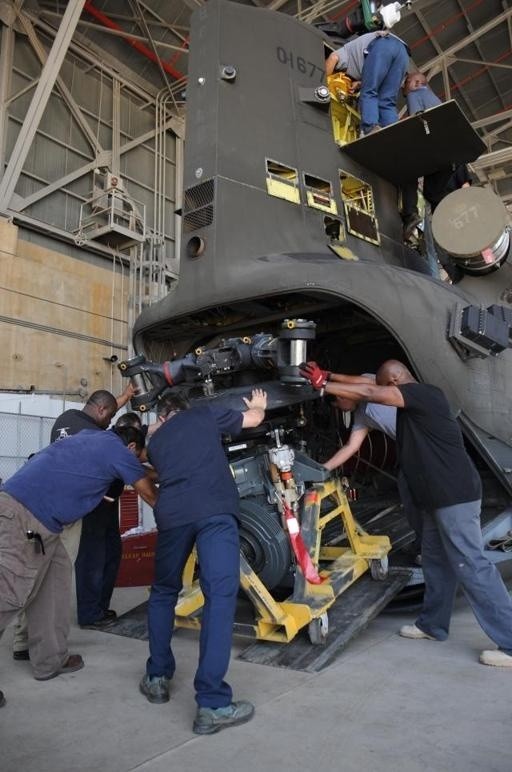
top-left (300, 359), bottom-right (512, 665)
top-left (326, 31), bottom-right (411, 137)
top-left (75, 413), bottom-right (165, 630)
top-left (13, 381), bottom-right (135, 661)
top-left (403, 73), bottom-right (442, 239)
top-left (321, 370), bottom-right (425, 565)
top-left (140, 388), bottom-right (268, 735)
top-left (0, 426), bottom-right (160, 708)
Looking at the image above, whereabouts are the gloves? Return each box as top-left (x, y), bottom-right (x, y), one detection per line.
top-left (299, 361), bottom-right (331, 394)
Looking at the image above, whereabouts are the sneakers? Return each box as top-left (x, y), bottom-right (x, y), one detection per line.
top-left (193, 700), bottom-right (255, 734)
top-left (367, 125), bottom-right (382, 135)
top-left (140, 674), bottom-right (170, 704)
top-left (399, 623), bottom-right (438, 640)
top-left (14, 649), bottom-right (30, 660)
top-left (34, 654), bottom-right (85, 681)
top-left (81, 610), bottom-right (117, 629)
top-left (479, 649), bottom-right (512, 666)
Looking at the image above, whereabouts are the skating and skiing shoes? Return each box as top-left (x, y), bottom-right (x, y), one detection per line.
top-left (0, 691), bottom-right (6, 708)
